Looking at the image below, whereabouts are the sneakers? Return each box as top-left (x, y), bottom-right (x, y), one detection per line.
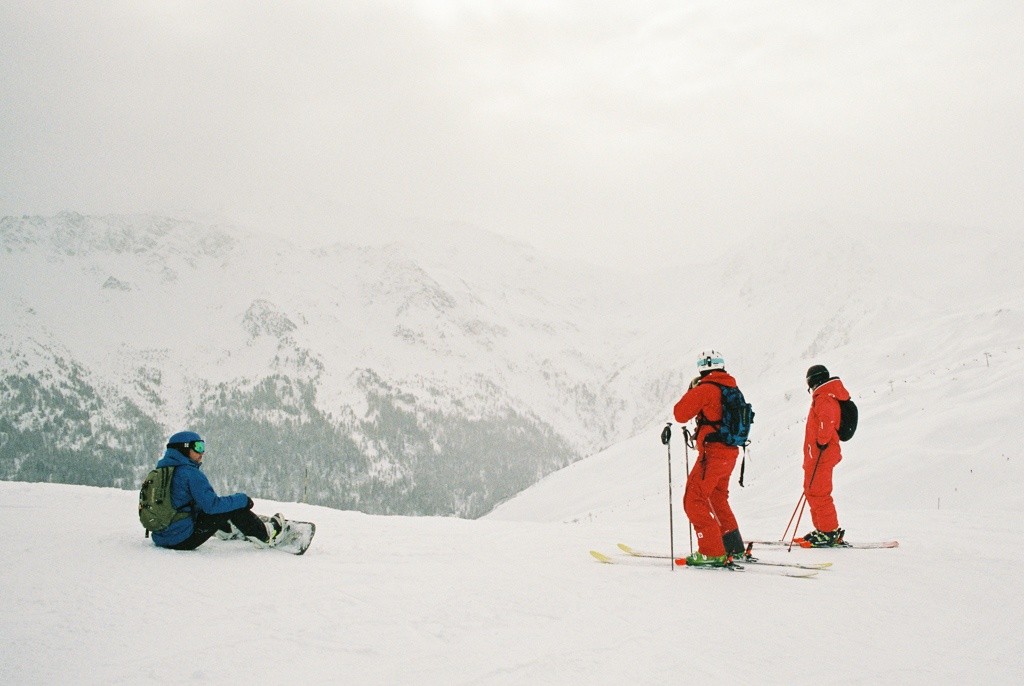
top-left (808, 532), bottom-right (839, 545)
top-left (685, 551), bottom-right (728, 567)
top-left (215, 520), bottom-right (242, 540)
top-left (803, 527), bottom-right (821, 541)
top-left (245, 513), bottom-right (286, 549)
top-left (726, 548), bottom-right (746, 560)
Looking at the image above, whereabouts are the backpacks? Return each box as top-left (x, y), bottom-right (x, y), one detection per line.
top-left (813, 396), bottom-right (859, 442)
top-left (138, 466), bottom-right (196, 539)
top-left (690, 380), bottom-right (756, 448)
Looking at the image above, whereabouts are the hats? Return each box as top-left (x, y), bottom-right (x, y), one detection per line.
top-left (169, 430), bottom-right (202, 457)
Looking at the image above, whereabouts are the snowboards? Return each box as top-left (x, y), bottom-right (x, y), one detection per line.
top-left (211, 514), bottom-right (316, 555)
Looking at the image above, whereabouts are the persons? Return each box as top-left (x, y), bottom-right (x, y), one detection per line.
top-left (153, 430), bottom-right (284, 550)
top-left (797, 364), bottom-right (849, 545)
top-left (674, 349), bottom-right (746, 566)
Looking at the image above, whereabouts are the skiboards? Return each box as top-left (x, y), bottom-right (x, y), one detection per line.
top-left (589, 542), bottom-right (833, 577)
top-left (742, 540), bottom-right (898, 549)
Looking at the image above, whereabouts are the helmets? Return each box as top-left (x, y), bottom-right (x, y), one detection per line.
top-left (806, 365), bottom-right (829, 388)
top-left (695, 349), bottom-right (725, 373)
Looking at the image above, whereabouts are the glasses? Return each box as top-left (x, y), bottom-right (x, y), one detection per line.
top-left (167, 440), bottom-right (206, 454)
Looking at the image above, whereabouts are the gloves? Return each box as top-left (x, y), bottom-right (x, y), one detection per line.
top-left (816, 440), bottom-right (828, 450)
top-left (687, 376), bottom-right (702, 390)
top-left (246, 495), bottom-right (255, 510)
